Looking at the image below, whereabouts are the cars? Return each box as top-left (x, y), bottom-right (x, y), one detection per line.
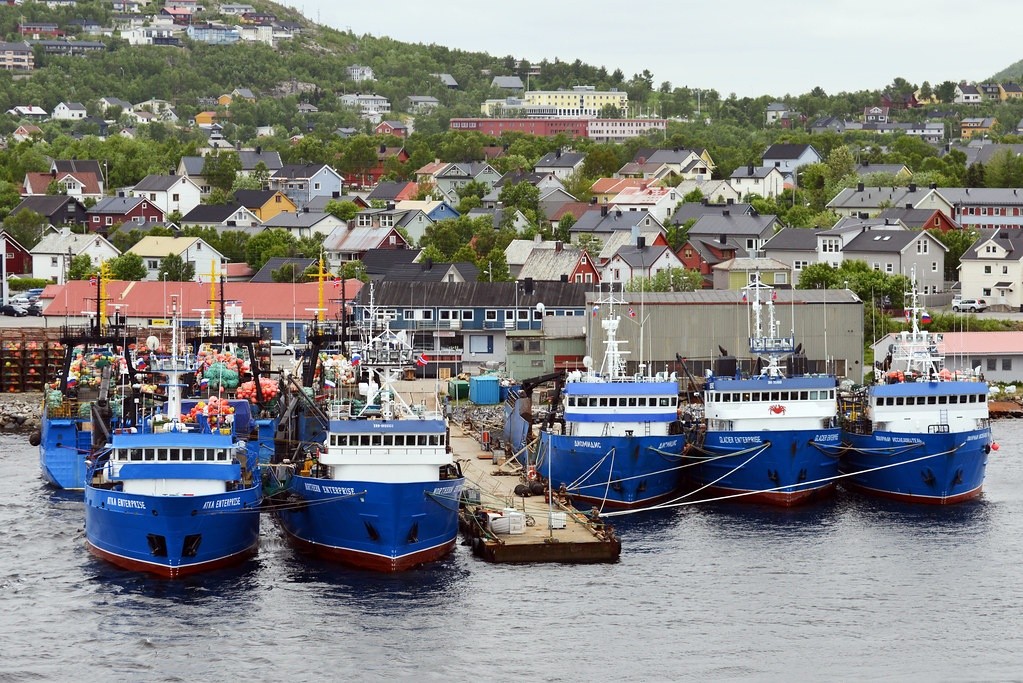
top-left (875, 299), bottom-right (892, 310)
top-left (264, 340), bottom-right (297, 356)
top-left (4, 287), bottom-right (43, 318)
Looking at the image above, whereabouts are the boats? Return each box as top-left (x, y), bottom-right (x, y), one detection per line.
top-left (671, 259), bottom-right (843, 507)
top-left (35, 233), bottom-right (418, 493)
top-left (819, 259), bottom-right (993, 507)
top-left (85, 258), bottom-right (266, 580)
top-left (503, 240), bottom-right (691, 512)
top-left (265, 280), bottom-right (465, 576)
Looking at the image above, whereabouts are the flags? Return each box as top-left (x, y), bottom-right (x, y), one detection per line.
top-left (628, 306), bottom-right (636, 317)
top-left (417, 353), bottom-right (429, 367)
top-left (592, 306), bottom-right (599, 317)
top-left (919, 311), bottom-right (932, 324)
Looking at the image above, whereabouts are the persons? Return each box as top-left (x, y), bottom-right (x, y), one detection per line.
top-left (444, 402), bottom-right (452, 418)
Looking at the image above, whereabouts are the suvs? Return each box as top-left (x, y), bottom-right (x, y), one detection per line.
top-left (952, 298), bottom-right (986, 314)
